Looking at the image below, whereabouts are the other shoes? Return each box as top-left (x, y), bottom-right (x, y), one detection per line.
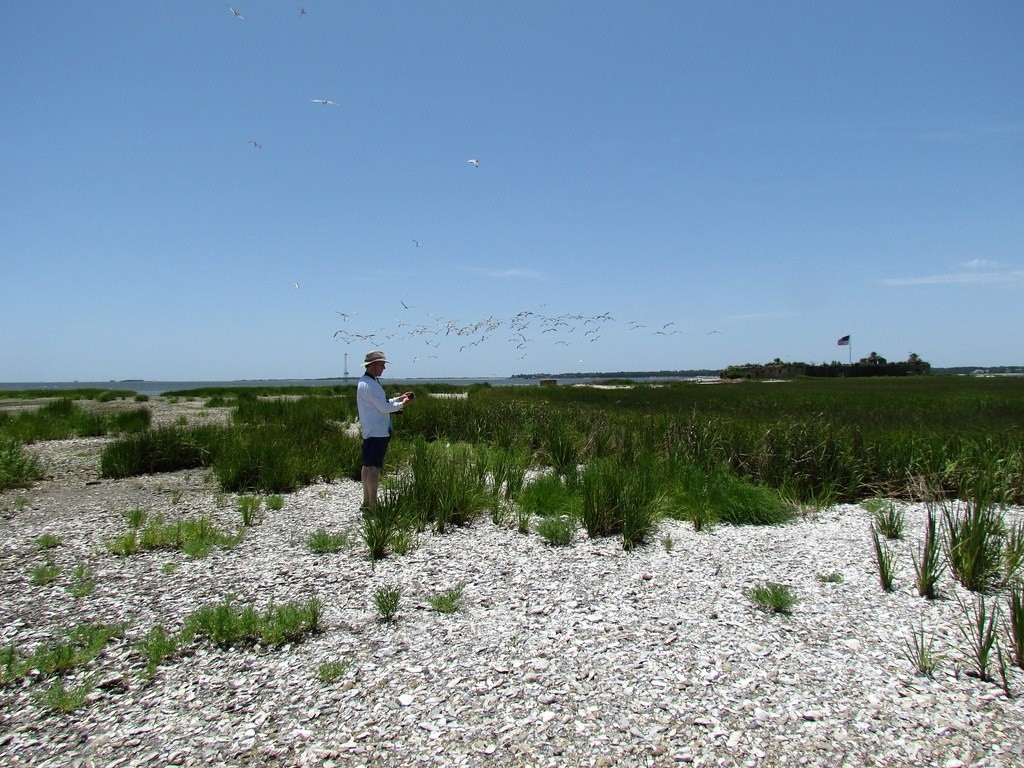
top-left (360, 504), bottom-right (368, 511)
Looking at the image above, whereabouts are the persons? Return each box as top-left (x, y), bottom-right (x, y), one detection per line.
top-left (357, 351), bottom-right (416, 512)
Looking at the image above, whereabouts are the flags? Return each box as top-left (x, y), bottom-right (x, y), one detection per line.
top-left (838, 335), bottom-right (851, 345)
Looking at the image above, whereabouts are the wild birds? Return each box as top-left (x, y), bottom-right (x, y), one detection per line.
top-left (228, 7), bottom-right (722, 364)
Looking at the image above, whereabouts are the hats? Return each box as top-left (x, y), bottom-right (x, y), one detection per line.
top-left (360, 350), bottom-right (389, 366)
